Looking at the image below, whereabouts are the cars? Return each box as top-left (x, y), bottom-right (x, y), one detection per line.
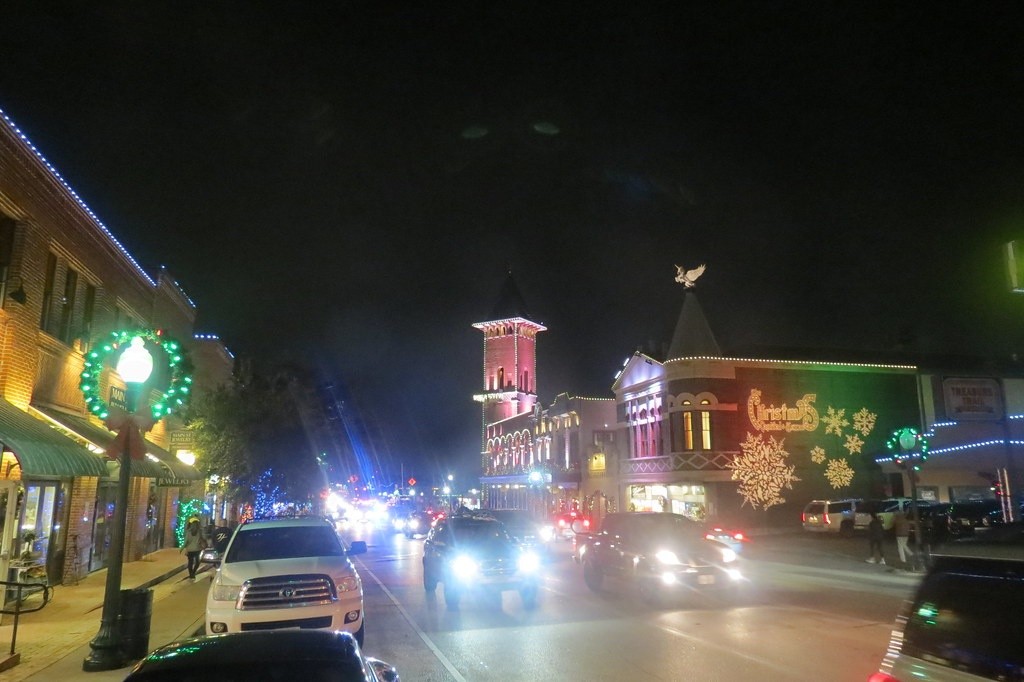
top-left (579, 509), bottom-right (741, 608)
top-left (330, 498), bottom-right (437, 541)
top-left (850, 498), bottom-right (914, 532)
top-left (866, 530), bottom-right (1024, 682)
top-left (693, 517), bottom-right (748, 556)
top-left (117, 625), bottom-right (399, 682)
top-left (901, 496), bottom-right (1024, 537)
top-left (800, 496), bottom-right (864, 534)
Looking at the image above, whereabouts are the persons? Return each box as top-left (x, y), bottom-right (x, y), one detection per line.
top-left (865, 512), bottom-right (887, 565)
top-left (211, 519), bottom-right (233, 569)
top-left (179, 520), bottom-right (206, 580)
top-left (892, 509), bottom-right (915, 565)
top-left (187, 513), bottom-right (201, 529)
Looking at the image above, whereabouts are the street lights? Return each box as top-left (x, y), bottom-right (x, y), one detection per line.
top-left (85, 338), bottom-right (158, 672)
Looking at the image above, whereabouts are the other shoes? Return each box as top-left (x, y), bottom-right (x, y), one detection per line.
top-left (865, 557), bottom-right (874, 564)
top-left (190, 575), bottom-right (196, 582)
top-left (878, 559), bottom-right (885, 566)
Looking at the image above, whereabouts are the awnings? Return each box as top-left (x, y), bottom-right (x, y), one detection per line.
top-left (0, 394), bottom-right (111, 480)
top-left (101, 422), bottom-right (204, 480)
top-left (27, 401), bottom-right (170, 478)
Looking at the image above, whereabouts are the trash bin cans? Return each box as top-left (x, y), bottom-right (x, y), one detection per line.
top-left (119, 587), bottom-right (155, 659)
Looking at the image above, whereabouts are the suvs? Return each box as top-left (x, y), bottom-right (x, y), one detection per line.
top-left (420, 513), bottom-right (538, 614)
top-left (199, 515), bottom-right (370, 647)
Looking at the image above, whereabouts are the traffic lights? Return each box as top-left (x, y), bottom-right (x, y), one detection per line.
top-left (993, 481), bottom-right (1002, 497)
